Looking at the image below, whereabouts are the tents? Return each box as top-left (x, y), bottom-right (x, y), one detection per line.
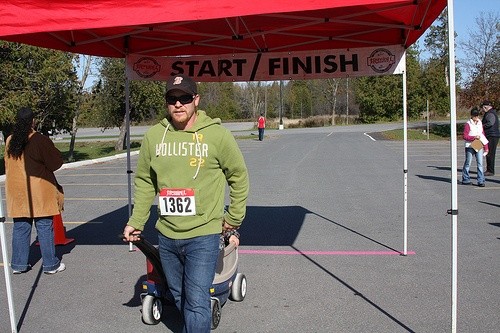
top-left (0, 0), bottom-right (461, 333)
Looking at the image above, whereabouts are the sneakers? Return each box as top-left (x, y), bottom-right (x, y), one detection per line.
top-left (13, 262), bottom-right (32, 274)
top-left (43, 262), bottom-right (65, 274)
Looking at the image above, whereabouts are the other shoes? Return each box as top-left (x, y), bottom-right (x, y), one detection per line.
top-left (483, 171), bottom-right (493, 175)
top-left (478, 183), bottom-right (485, 187)
top-left (462, 181), bottom-right (472, 185)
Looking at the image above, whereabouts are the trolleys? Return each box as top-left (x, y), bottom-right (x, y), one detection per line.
top-left (118, 225), bottom-right (247, 329)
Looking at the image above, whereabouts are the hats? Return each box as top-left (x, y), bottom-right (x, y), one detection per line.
top-left (471, 108), bottom-right (480, 115)
top-left (165, 74), bottom-right (197, 96)
top-left (480, 101), bottom-right (492, 108)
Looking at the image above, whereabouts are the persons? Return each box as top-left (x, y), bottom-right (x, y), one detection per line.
top-left (479, 100), bottom-right (500, 176)
top-left (4, 107), bottom-right (66, 274)
top-left (123, 74), bottom-right (250, 333)
top-left (256, 113), bottom-right (266, 141)
top-left (461, 107), bottom-right (489, 187)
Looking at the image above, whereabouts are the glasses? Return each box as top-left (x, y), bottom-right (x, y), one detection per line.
top-left (166, 93), bottom-right (195, 105)
